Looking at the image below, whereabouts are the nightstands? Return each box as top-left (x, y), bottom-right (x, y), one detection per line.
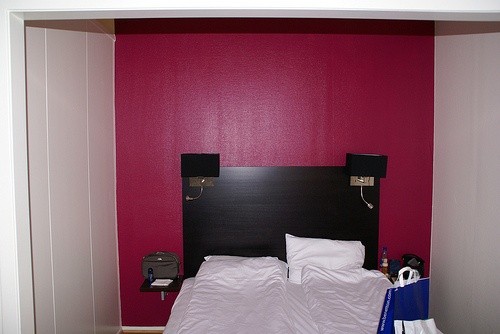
top-left (139, 276), bottom-right (179, 296)
top-left (385, 266), bottom-right (427, 283)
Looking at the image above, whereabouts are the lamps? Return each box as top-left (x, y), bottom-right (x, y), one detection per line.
top-left (346, 152), bottom-right (388, 209)
top-left (181, 152), bottom-right (220, 202)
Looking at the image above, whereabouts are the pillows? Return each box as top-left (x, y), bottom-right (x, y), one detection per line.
top-left (204, 255), bottom-right (287, 285)
top-left (284, 233), bottom-right (368, 284)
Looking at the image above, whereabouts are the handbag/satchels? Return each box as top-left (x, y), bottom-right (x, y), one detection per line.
top-left (394, 317), bottom-right (438, 334)
top-left (401, 254), bottom-right (425, 280)
top-left (376, 266), bottom-right (429, 334)
top-left (142, 251), bottom-right (180, 279)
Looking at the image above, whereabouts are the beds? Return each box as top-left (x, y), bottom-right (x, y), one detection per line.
top-left (162, 167), bottom-right (446, 334)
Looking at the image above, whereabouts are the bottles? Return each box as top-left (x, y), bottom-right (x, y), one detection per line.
top-left (382, 258), bottom-right (388, 273)
top-left (380, 247), bottom-right (388, 270)
